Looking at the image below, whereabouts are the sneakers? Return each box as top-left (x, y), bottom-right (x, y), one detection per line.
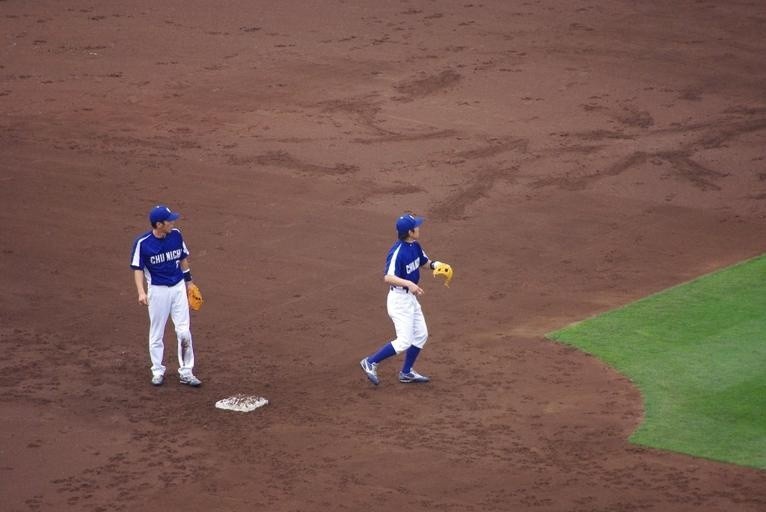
top-left (180, 376), bottom-right (202, 386)
top-left (152, 371), bottom-right (164, 384)
top-left (360, 357), bottom-right (379, 385)
top-left (399, 368), bottom-right (429, 383)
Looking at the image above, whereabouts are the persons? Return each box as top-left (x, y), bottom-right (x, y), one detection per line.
top-left (359, 214), bottom-right (454, 385)
top-left (130, 205), bottom-right (203, 387)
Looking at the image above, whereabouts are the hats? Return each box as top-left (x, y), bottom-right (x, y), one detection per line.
top-left (150, 205), bottom-right (180, 223)
top-left (396, 214), bottom-right (424, 233)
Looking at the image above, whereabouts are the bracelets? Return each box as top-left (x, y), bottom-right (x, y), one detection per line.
top-left (181, 268), bottom-right (192, 283)
top-left (429, 260), bottom-right (439, 270)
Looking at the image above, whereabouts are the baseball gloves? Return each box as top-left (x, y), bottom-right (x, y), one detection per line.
top-left (433, 262), bottom-right (453, 287)
top-left (187, 282), bottom-right (203, 311)
